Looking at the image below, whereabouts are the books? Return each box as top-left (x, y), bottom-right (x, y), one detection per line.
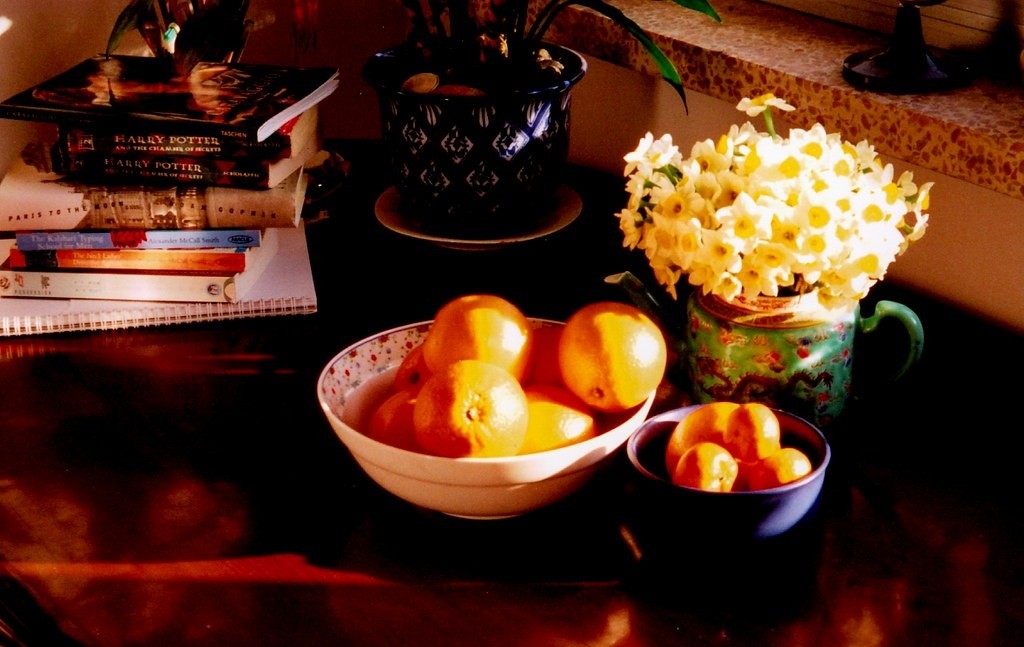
top-left (0, 52), bottom-right (341, 336)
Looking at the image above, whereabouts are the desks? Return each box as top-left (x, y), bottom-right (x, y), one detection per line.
top-left (0, 136), bottom-right (1024, 646)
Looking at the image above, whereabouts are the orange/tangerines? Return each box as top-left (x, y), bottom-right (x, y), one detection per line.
top-left (367, 294), bottom-right (666, 460)
top-left (666, 399), bottom-right (811, 493)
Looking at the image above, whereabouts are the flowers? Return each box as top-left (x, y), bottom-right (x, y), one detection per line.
top-left (614, 93), bottom-right (935, 314)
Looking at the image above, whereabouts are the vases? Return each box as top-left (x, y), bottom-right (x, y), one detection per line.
top-left (605, 267), bottom-right (926, 431)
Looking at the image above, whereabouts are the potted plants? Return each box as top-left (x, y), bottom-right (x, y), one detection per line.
top-left (356, 0), bottom-right (723, 242)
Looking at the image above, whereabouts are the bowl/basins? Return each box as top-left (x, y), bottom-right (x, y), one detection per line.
top-left (626, 404), bottom-right (831, 545)
top-left (317, 317), bottom-right (656, 520)
